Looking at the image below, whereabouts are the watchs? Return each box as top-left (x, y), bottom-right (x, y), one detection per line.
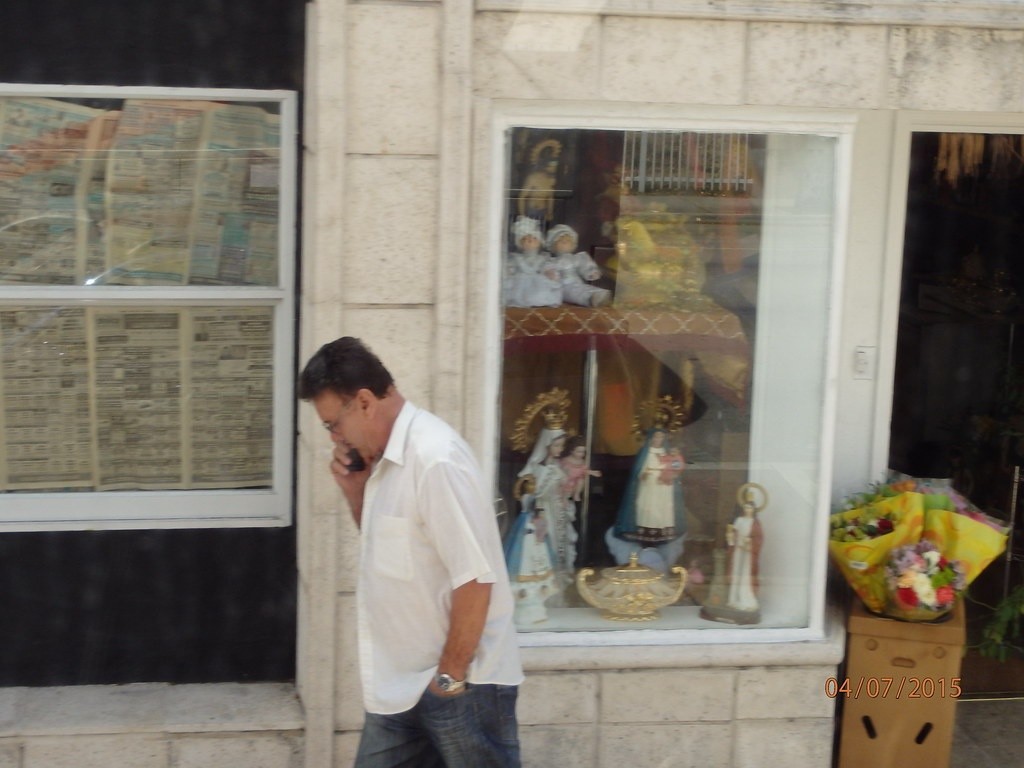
top-left (434, 673), bottom-right (467, 691)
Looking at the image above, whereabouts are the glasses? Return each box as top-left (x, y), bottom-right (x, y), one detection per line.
top-left (322, 397), bottom-right (354, 432)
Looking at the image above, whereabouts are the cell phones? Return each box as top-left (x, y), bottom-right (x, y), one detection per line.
top-left (341, 444), bottom-right (366, 472)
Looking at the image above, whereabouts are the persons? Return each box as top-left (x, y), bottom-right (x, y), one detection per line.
top-left (507, 215), bottom-right (563, 307)
top-left (726, 500), bottom-right (764, 611)
top-left (547, 224), bottom-right (612, 307)
top-left (612, 428), bottom-right (687, 546)
top-left (517, 156), bottom-right (559, 221)
top-left (297, 336), bottom-right (524, 768)
top-left (502, 429), bottom-right (601, 581)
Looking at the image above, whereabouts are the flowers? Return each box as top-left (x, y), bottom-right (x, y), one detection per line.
top-left (822, 466), bottom-right (1013, 623)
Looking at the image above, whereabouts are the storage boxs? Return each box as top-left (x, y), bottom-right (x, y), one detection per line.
top-left (838, 594), bottom-right (966, 768)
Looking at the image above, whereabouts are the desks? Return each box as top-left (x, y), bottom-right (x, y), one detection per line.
top-left (503, 300), bottom-right (750, 573)
